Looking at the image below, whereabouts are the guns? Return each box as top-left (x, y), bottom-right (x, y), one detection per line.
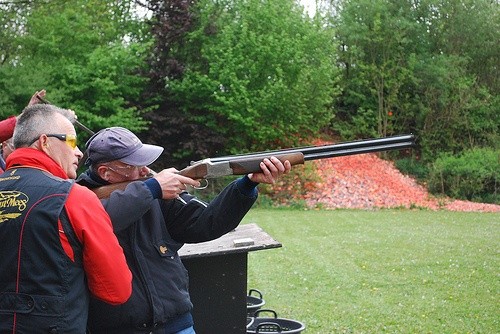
top-left (90, 133), bottom-right (419, 207)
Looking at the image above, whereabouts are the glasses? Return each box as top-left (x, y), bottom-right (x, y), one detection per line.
top-left (100, 165), bottom-right (142, 177)
top-left (28, 134), bottom-right (77, 150)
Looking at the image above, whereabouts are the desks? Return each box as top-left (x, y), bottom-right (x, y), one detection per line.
top-left (177, 223), bottom-right (282, 334)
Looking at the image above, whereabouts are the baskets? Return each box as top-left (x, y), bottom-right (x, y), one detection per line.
top-left (246, 288), bottom-right (264, 317)
top-left (245, 309), bottom-right (305, 334)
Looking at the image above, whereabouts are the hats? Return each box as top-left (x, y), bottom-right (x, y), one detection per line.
top-left (84, 126), bottom-right (164, 166)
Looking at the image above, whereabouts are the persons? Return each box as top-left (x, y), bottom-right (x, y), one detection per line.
top-left (0, 89), bottom-right (77, 174)
top-left (70, 125), bottom-right (292, 334)
top-left (0, 105), bottom-right (134, 333)
